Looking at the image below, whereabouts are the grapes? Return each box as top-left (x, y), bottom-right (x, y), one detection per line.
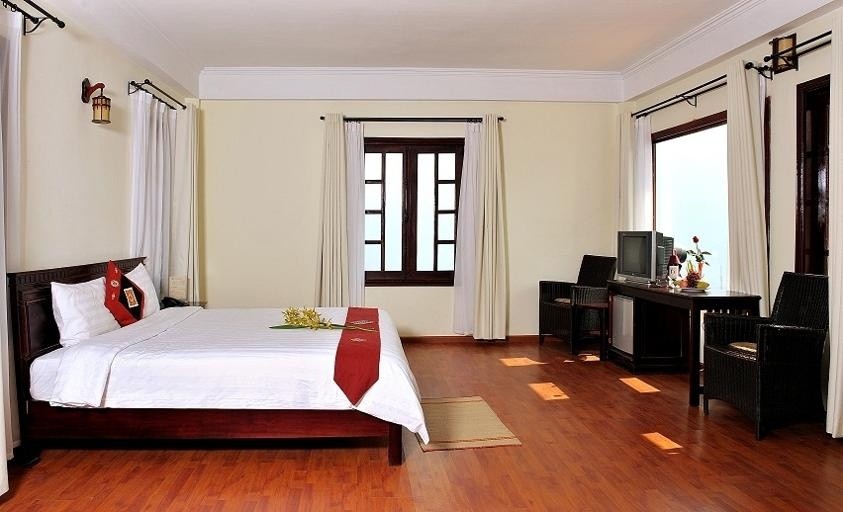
top-left (686, 269), bottom-right (700, 288)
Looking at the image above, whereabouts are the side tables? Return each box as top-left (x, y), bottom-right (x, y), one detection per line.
top-left (573, 303), bottom-right (608, 361)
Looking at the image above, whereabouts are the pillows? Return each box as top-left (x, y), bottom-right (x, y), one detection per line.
top-left (50, 261), bottom-right (161, 348)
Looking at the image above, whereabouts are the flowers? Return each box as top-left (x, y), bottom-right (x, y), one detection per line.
top-left (269, 305), bottom-right (380, 333)
top-left (686, 236), bottom-right (712, 265)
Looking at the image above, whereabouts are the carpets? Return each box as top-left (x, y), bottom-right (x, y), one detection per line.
top-left (414, 395), bottom-right (522, 452)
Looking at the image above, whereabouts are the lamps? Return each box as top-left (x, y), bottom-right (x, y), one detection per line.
top-left (81, 78), bottom-right (111, 124)
top-left (772, 33), bottom-right (797, 74)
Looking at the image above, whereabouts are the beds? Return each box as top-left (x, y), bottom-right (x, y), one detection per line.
top-left (3, 257), bottom-right (403, 466)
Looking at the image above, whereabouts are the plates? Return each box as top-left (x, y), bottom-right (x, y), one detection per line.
top-left (682, 288), bottom-right (705, 293)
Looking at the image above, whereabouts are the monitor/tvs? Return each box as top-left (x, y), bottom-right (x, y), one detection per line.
top-left (616, 230), bottom-right (673, 285)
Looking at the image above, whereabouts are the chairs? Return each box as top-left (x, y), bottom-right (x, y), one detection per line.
top-left (704, 271), bottom-right (828, 440)
top-left (539, 255), bottom-right (617, 355)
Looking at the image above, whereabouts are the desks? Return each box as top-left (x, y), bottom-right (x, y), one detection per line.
top-left (606, 280), bottom-right (761, 406)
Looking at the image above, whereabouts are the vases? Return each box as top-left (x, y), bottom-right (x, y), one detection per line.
top-left (697, 263), bottom-right (704, 278)
